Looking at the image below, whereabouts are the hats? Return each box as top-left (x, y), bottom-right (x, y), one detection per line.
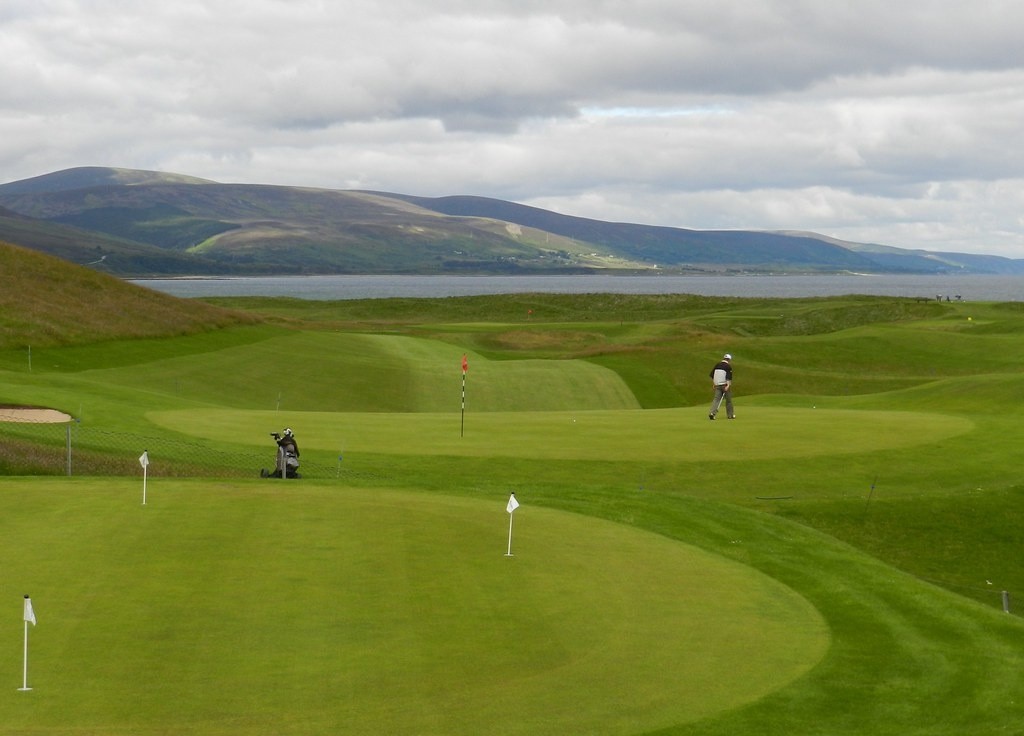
top-left (724, 354), bottom-right (732, 360)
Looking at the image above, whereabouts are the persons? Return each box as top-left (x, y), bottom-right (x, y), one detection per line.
top-left (260, 429), bottom-right (302, 479)
top-left (709, 354), bottom-right (736, 421)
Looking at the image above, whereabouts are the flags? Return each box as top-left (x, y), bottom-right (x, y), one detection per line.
top-left (24, 598), bottom-right (36, 625)
top-left (462, 356), bottom-right (468, 371)
top-left (339, 455), bottom-right (343, 460)
top-left (138, 453), bottom-right (149, 468)
top-left (506, 494), bottom-right (519, 514)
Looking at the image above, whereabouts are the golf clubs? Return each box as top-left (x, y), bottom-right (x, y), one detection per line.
top-left (711, 392), bottom-right (726, 419)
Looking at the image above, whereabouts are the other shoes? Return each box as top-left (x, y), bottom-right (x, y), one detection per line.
top-left (709, 413), bottom-right (716, 420)
top-left (729, 415), bottom-right (736, 419)
